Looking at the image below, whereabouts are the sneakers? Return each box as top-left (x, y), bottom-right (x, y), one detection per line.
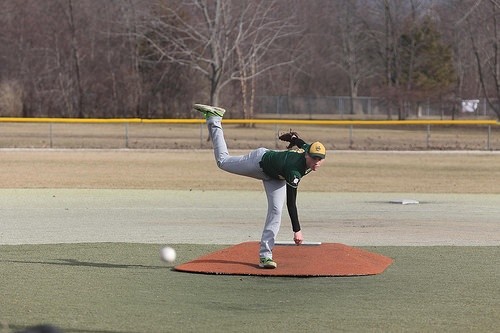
top-left (192, 103), bottom-right (226, 117)
top-left (259, 258), bottom-right (276, 268)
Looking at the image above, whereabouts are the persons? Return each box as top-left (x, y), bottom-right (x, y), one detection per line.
top-left (193, 104), bottom-right (326, 269)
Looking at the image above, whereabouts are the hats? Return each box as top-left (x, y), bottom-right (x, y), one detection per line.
top-left (308, 141), bottom-right (325, 159)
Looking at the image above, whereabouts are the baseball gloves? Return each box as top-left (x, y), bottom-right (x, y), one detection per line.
top-left (278, 128), bottom-right (300, 149)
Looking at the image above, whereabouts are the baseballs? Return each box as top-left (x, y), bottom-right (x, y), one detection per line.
top-left (160, 246), bottom-right (176, 262)
